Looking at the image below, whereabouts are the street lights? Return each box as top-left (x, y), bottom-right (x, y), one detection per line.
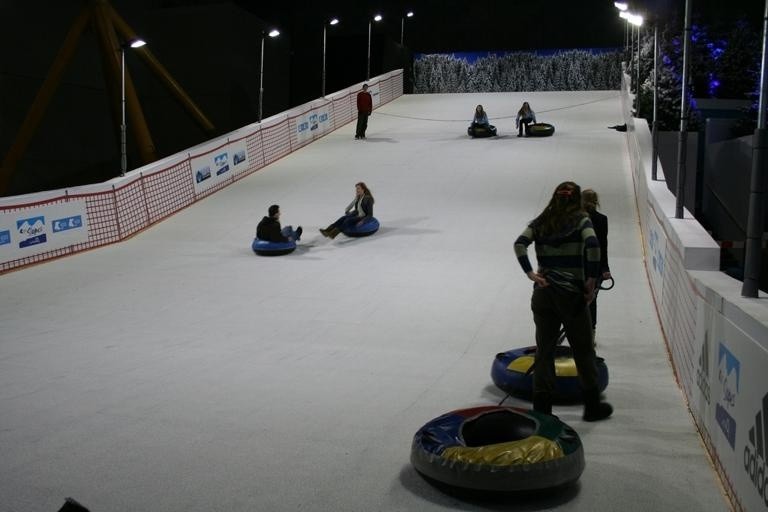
top-left (318, 12), bottom-right (339, 98)
top-left (398, 7), bottom-right (414, 74)
top-left (363, 10), bottom-right (383, 82)
top-left (612, 1), bottom-right (662, 184)
top-left (111, 35), bottom-right (154, 176)
top-left (256, 25), bottom-right (281, 124)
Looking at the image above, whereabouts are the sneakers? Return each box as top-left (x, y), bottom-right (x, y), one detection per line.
top-left (296, 226), bottom-right (302, 237)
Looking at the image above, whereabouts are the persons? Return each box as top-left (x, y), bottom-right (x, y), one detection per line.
top-left (516, 102), bottom-right (536, 137)
top-left (355, 84), bottom-right (372, 140)
top-left (579, 189), bottom-right (611, 348)
top-left (257, 205), bottom-right (302, 243)
top-left (470, 105), bottom-right (493, 139)
top-left (514, 182), bottom-right (613, 421)
top-left (320, 183), bottom-right (374, 239)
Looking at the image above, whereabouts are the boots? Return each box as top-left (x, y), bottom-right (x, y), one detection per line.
top-left (583, 391), bottom-right (613, 422)
top-left (535, 392), bottom-right (559, 419)
top-left (319, 226), bottom-right (340, 239)
